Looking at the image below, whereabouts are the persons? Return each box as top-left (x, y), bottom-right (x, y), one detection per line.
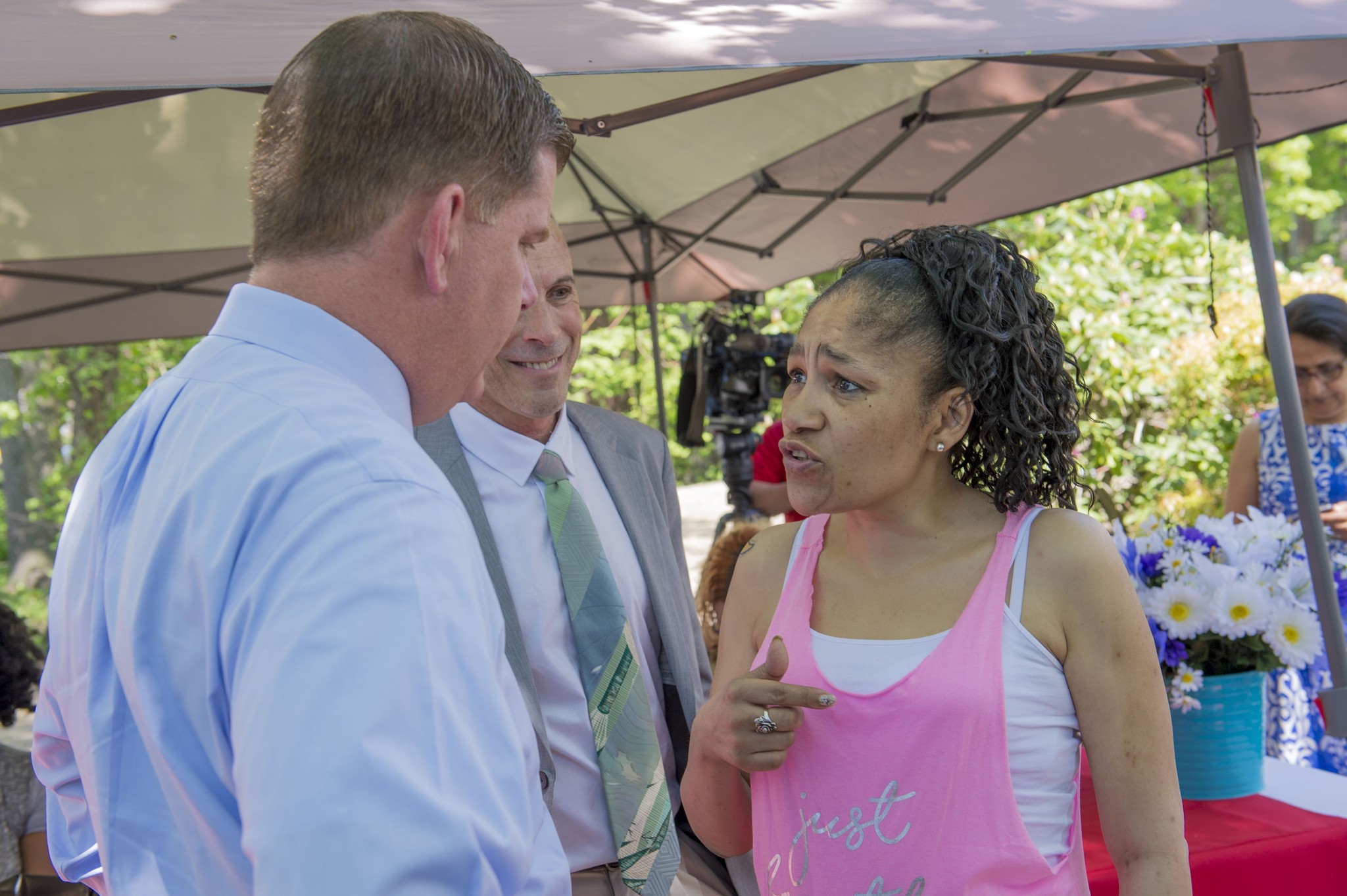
top-left (1225, 294), bottom-right (1347, 775)
top-left (693, 520), bottom-right (756, 653)
top-left (419, 218), bottom-right (748, 895)
top-left (680, 225), bottom-right (1192, 896)
top-left (0, 603), bottom-right (63, 880)
top-left (749, 419), bottom-right (813, 524)
top-left (29, 10), bottom-right (578, 896)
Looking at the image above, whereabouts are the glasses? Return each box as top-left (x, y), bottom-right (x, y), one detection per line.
top-left (1297, 357), bottom-right (1347, 389)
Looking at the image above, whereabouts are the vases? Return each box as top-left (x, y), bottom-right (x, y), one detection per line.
top-left (1162, 671), bottom-right (1267, 800)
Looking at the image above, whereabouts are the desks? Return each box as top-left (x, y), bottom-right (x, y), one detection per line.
top-left (1077, 739), bottom-right (1347, 896)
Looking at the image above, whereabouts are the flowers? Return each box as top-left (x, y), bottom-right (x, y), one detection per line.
top-left (1102, 504), bottom-right (1325, 713)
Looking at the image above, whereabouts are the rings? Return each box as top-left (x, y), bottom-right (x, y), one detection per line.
top-left (754, 708), bottom-right (777, 733)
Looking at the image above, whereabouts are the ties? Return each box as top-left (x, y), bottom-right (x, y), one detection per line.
top-left (531, 445), bottom-right (681, 896)
